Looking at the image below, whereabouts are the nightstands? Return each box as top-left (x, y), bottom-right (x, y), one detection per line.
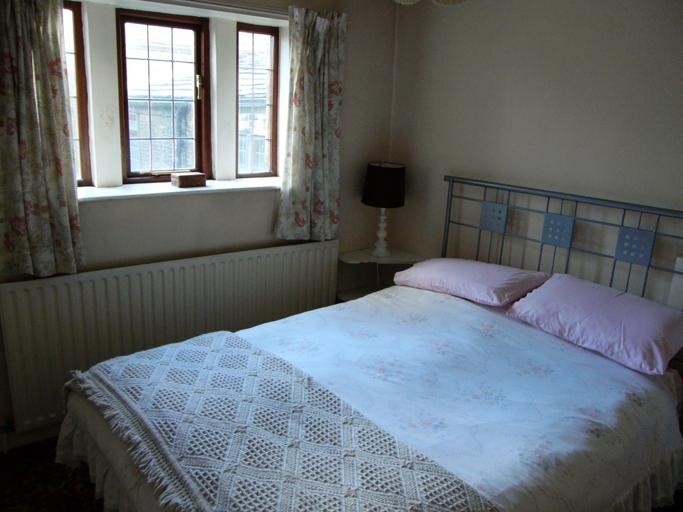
top-left (337, 248), bottom-right (427, 305)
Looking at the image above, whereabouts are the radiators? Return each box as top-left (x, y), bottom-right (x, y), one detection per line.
top-left (2, 234), bottom-right (340, 434)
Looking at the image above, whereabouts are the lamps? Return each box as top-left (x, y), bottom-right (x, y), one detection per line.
top-left (359, 160), bottom-right (406, 257)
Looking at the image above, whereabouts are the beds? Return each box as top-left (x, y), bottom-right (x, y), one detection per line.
top-left (62, 174), bottom-right (682, 512)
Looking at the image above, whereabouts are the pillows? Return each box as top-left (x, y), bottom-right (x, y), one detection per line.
top-left (393, 255), bottom-right (681, 376)
